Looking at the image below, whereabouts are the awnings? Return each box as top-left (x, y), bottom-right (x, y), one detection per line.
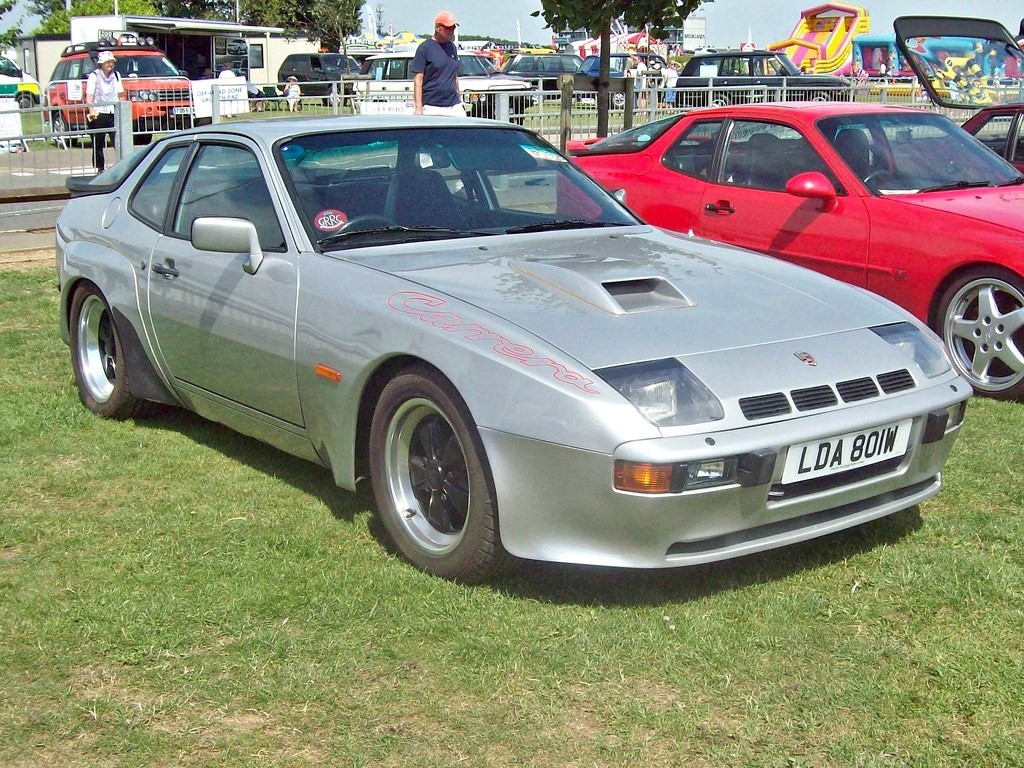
top-left (128, 20), bottom-right (284, 35)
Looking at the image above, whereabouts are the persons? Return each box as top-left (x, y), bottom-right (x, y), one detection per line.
top-left (659, 68), bottom-right (678, 113)
top-left (920, 69), bottom-right (933, 111)
top-left (283, 76), bottom-right (301, 112)
top-left (86, 50), bottom-right (125, 173)
top-left (887, 68), bottom-right (892, 84)
top-left (950, 75), bottom-right (962, 104)
top-left (412, 12), bottom-right (467, 118)
top-left (200, 56), bottom-right (265, 112)
top-left (878, 63), bottom-right (886, 83)
top-left (625, 50), bottom-right (648, 116)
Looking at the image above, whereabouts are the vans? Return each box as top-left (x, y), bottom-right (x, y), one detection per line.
top-left (0, 54), bottom-right (43, 108)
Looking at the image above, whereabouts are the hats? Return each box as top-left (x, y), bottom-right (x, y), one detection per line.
top-left (96, 51), bottom-right (117, 63)
top-left (288, 76), bottom-right (298, 82)
top-left (436, 11), bottom-right (460, 27)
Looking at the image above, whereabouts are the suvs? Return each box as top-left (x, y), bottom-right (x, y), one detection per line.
top-left (277, 52), bottom-right (362, 107)
top-left (672, 46), bottom-right (856, 108)
top-left (42, 35), bottom-right (199, 149)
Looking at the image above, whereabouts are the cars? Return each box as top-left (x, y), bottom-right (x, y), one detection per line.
top-left (556, 15), bottom-right (1024, 402)
top-left (53, 112), bottom-right (975, 587)
top-left (501, 46), bottom-right (682, 108)
top-left (356, 51), bottom-right (535, 127)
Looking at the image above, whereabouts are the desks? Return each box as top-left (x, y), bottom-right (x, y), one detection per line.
top-left (139, 60), bottom-right (158, 75)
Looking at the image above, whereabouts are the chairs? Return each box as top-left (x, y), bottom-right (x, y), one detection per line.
top-left (399, 169), bottom-right (471, 232)
top-left (747, 131), bottom-right (814, 193)
top-left (263, 87), bottom-right (283, 111)
top-left (753, 67), bottom-right (763, 76)
top-left (389, 68), bottom-right (403, 79)
top-left (833, 128), bottom-right (872, 184)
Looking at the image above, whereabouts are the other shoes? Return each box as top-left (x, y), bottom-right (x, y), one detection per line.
top-left (97, 169), bottom-right (105, 174)
